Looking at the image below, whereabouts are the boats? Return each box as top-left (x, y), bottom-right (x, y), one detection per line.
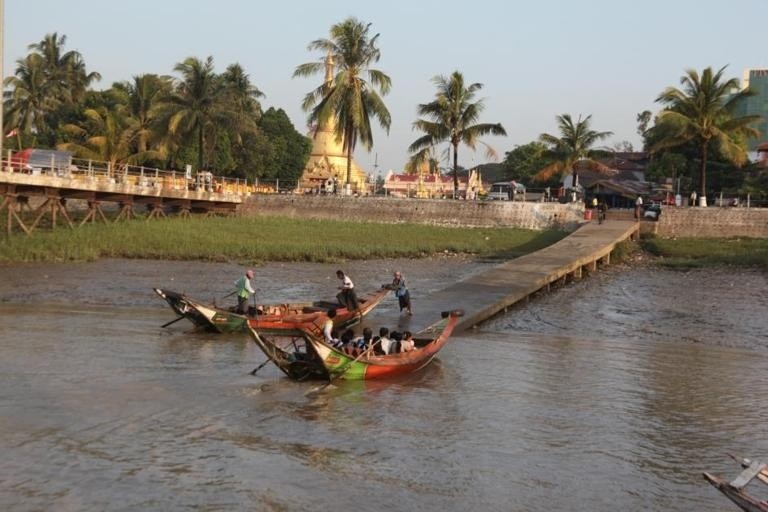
top-left (151, 280), bottom-right (393, 339)
top-left (245, 310), bottom-right (457, 384)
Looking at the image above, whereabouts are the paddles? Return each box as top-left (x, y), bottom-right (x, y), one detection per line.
top-left (252, 292), bottom-right (261, 335)
top-left (316, 337), bottom-right (383, 392)
top-left (248, 325), bottom-right (320, 376)
top-left (161, 290), bottom-right (240, 329)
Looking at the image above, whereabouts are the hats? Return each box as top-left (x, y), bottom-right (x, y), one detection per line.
top-left (247, 271), bottom-right (254, 279)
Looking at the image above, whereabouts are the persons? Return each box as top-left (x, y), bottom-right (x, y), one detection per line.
top-left (335, 269), bottom-right (363, 322)
top-left (194, 168), bottom-right (217, 190)
top-left (690, 189), bottom-right (698, 207)
top-left (233, 270), bottom-right (257, 315)
top-left (558, 182), bottom-right (565, 201)
top-left (392, 270), bottom-right (413, 316)
top-left (675, 191), bottom-right (682, 208)
top-left (304, 172), bottom-right (340, 196)
top-left (508, 187), bottom-right (514, 201)
top-left (634, 194), bottom-right (644, 219)
top-left (470, 186), bottom-right (476, 200)
top-left (305, 306), bottom-right (417, 354)
top-left (602, 200), bottom-right (608, 220)
top-left (543, 183), bottom-right (551, 203)
top-left (596, 199), bottom-right (603, 224)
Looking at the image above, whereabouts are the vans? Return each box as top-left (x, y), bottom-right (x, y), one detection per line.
top-left (482, 181), bottom-right (526, 200)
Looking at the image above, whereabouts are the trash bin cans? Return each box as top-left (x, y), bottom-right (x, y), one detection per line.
top-left (585, 209), bottom-right (593, 220)
